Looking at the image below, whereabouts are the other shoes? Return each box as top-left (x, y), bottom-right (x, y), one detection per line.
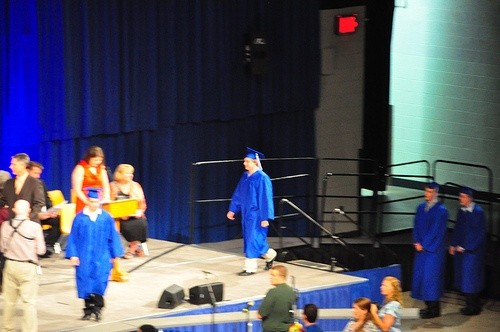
top-left (124, 252), bottom-right (135, 259)
top-left (135, 245), bottom-right (144, 253)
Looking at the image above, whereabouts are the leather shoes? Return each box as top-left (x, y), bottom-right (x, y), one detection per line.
top-left (265, 261), bottom-right (273, 270)
top-left (96, 313), bottom-right (101, 319)
top-left (462, 307), bottom-right (480, 314)
top-left (420, 308), bottom-right (429, 314)
top-left (422, 312), bottom-right (439, 319)
top-left (239, 271), bottom-right (255, 275)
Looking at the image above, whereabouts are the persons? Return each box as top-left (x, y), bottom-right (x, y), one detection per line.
top-left (71, 146), bottom-right (110, 215)
top-left (109, 164), bottom-right (149, 258)
top-left (289, 304), bottom-right (325, 332)
top-left (343, 298), bottom-right (382, 332)
top-left (0, 200), bottom-right (46, 332)
top-left (0, 153), bottom-right (62, 293)
top-left (449, 186), bottom-right (487, 315)
top-left (370, 276), bottom-right (403, 332)
top-left (64, 188), bottom-right (124, 322)
top-left (257, 265), bottom-right (297, 332)
top-left (137, 324), bottom-right (158, 332)
top-left (412, 181), bottom-right (450, 318)
top-left (227, 147), bottom-right (277, 276)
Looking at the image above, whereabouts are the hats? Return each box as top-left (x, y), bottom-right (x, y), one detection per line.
top-left (461, 185), bottom-right (474, 196)
top-left (426, 181), bottom-right (440, 202)
top-left (246, 147), bottom-right (264, 170)
top-left (83, 189), bottom-right (102, 198)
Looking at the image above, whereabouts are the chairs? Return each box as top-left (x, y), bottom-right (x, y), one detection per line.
top-left (46, 191), bottom-right (75, 235)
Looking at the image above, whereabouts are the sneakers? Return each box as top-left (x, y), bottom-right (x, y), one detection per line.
top-left (83, 314), bottom-right (91, 320)
